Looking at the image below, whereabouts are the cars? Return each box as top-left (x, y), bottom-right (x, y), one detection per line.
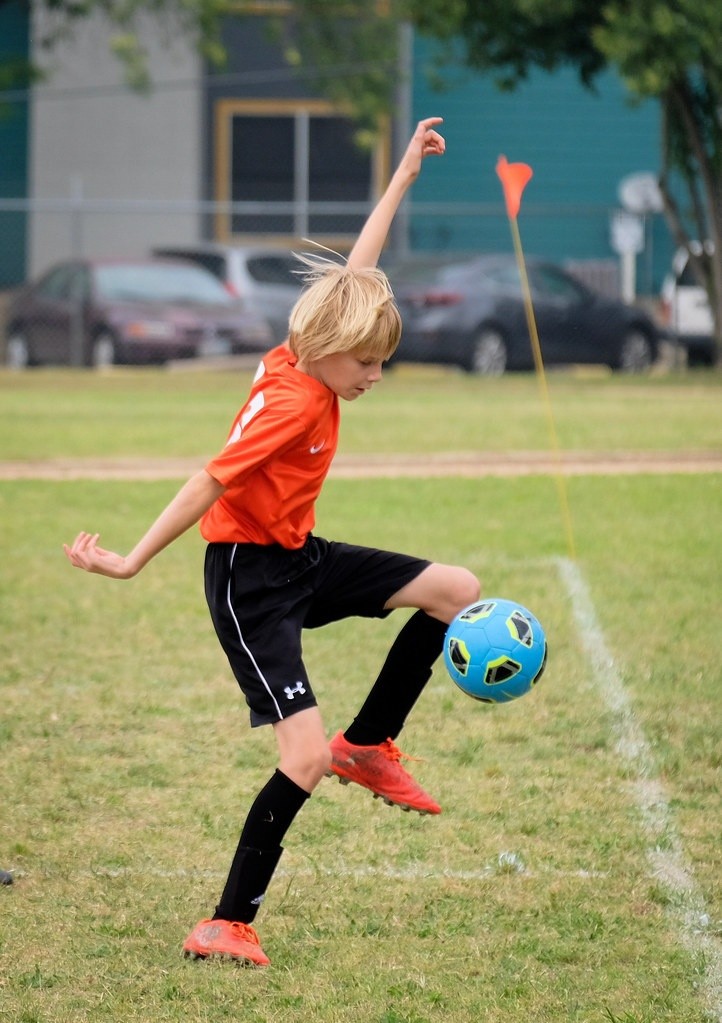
top-left (147, 240), bottom-right (341, 354)
top-left (0, 250), bottom-right (271, 372)
top-left (374, 250), bottom-right (666, 379)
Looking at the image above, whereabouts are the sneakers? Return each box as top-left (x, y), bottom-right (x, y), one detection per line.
top-left (323, 729), bottom-right (442, 816)
top-left (181, 918), bottom-right (272, 968)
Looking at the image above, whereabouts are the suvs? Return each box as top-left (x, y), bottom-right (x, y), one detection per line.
top-left (655, 239), bottom-right (722, 373)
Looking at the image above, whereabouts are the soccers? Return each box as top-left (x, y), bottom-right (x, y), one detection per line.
top-left (440, 595), bottom-right (552, 707)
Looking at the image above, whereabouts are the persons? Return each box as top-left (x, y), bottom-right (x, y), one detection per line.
top-left (63, 116), bottom-right (481, 966)
